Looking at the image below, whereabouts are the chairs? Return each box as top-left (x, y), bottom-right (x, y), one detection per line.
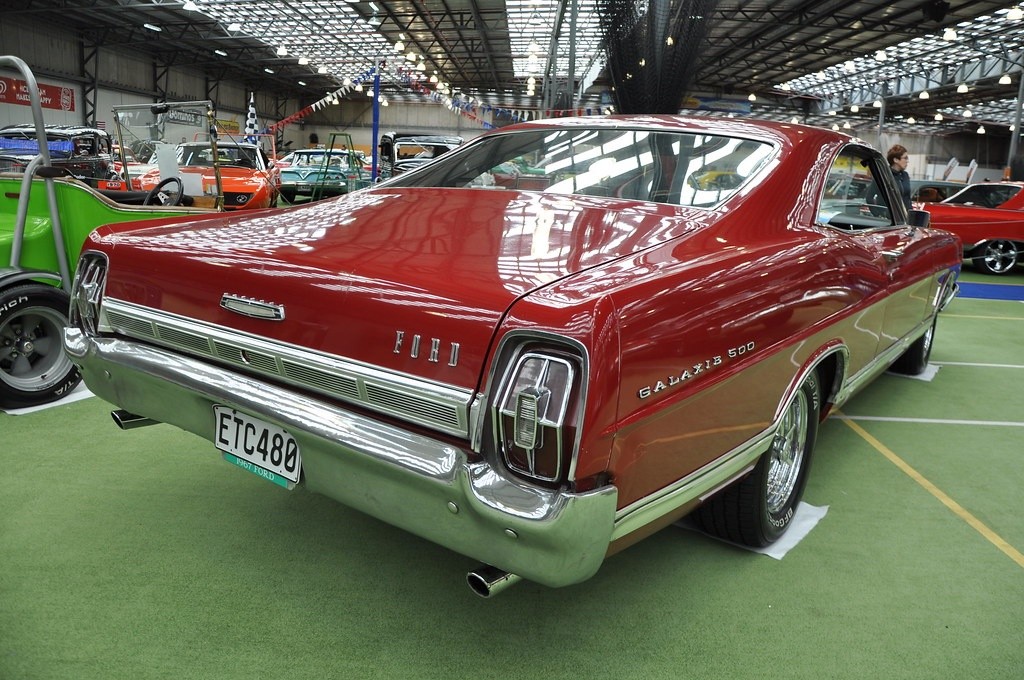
top-left (919, 188), bottom-right (938, 202)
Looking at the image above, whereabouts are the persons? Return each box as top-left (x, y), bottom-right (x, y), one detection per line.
top-left (866, 145), bottom-right (912, 218)
top-left (179, 137), bottom-right (187, 145)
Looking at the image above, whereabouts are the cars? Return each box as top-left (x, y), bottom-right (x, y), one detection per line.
top-left (908, 179), bottom-right (995, 206)
top-left (491, 158), bottom-right (547, 175)
top-left (59, 113), bottom-right (961, 592)
top-left (907, 182), bottom-right (1023, 276)
top-left (827, 173), bottom-right (877, 201)
top-left (139, 132), bottom-right (281, 212)
top-left (280, 148), bottom-right (372, 204)
top-left (0, 54), bottom-right (232, 411)
top-left (98, 139), bottom-right (170, 190)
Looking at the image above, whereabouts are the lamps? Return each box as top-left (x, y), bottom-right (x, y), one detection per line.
top-left (182, 0), bottom-right (1024, 135)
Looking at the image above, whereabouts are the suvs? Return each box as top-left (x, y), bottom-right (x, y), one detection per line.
top-left (375, 132), bottom-right (464, 188)
top-left (0, 123), bottom-right (116, 188)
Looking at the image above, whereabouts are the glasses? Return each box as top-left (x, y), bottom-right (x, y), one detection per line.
top-left (900, 156), bottom-right (908, 160)
